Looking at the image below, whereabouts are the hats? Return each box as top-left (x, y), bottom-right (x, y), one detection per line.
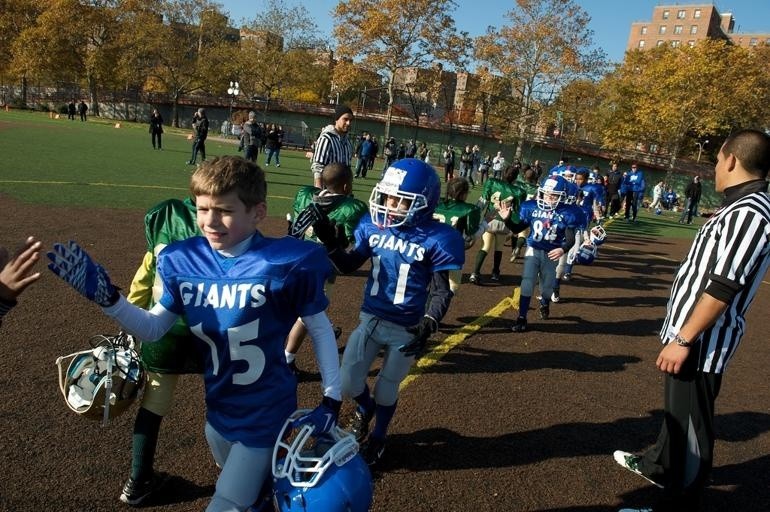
top-left (334, 105), bottom-right (353, 121)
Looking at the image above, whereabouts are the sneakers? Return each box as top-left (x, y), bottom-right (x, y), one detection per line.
top-left (470, 251), bottom-right (572, 332)
top-left (152, 144), bottom-right (282, 169)
top-left (363, 434), bottom-right (386, 468)
top-left (119, 473), bottom-right (154, 505)
top-left (613, 450), bottom-right (668, 488)
top-left (608, 206), bottom-right (701, 228)
top-left (347, 398), bottom-right (373, 441)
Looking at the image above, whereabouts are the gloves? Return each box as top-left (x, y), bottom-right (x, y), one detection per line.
top-left (44, 239), bottom-right (117, 309)
top-left (398, 317), bottom-right (438, 359)
top-left (293, 397), bottom-right (339, 440)
top-left (312, 188), bottom-right (341, 224)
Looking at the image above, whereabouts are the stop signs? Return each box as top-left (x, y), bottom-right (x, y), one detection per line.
top-left (553, 130), bottom-right (559, 135)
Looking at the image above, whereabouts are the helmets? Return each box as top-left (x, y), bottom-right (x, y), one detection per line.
top-left (274, 408), bottom-right (376, 510)
top-left (576, 241), bottom-right (598, 264)
top-left (590, 223), bottom-right (607, 245)
top-left (53, 336), bottom-right (138, 421)
top-left (366, 156), bottom-right (440, 229)
top-left (536, 176), bottom-right (568, 211)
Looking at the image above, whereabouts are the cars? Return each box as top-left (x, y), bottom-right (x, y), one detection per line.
top-left (533, 136), bottom-right (548, 145)
top-left (220, 117), bottom-right (246, 140)
top-left (317, 107), bottom-right (481, 136)
top-left (0, 85), bottom-right (215, 106)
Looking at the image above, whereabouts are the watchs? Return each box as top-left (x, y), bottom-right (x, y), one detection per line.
top-left (675, 335), bottom-right (691, 348)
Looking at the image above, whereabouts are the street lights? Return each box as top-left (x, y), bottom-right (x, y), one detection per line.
top-left (226, 79), bottom-right (239, 123)
top-left (693, 138), bottom-right (709, 166)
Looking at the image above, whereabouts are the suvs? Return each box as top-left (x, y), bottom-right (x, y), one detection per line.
top-left (251, 96), bottom-right (278, 106)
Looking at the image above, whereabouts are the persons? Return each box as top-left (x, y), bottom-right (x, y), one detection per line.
top-left (679, 175), bottom-right (701, 225)
top-left (46, 156), bottom-right (342, 512)
top-left (308, 157), bottom-right (465, 467)
top-left (69, 101), bottom-right (75, 120)
top-left (120, 198), bottom-right (214, 505)
top-left (186, 108), bottom-right (209, 165)
top-left (614, 131), bottom-right (770, 512)
top-left (149, 107), bottom-right (163, 151)
top-left (0, 236), bottom-right (42, 328)
top-left (238, 111), bottom-right (325, 167)
top-left (80, 101), bottom-right (88, 121)
top-left (284, 163), bottom-right (370, 382)
top-left (311, 106), bottom-right (357, 276)
